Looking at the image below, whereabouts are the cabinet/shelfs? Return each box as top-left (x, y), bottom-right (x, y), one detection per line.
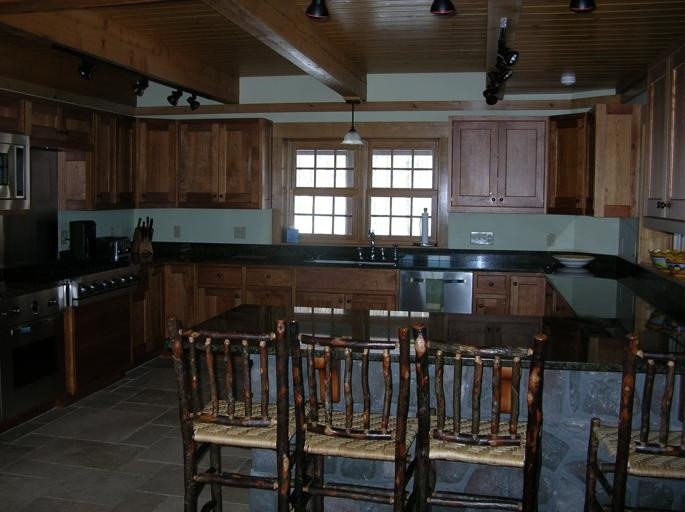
top-left (296, 291), bottom-right (397, 310)
top-left (477, 275), bottom-right (506, 316)
top-left (544, 281), bottom-right (570, 316)
top-left (148, 264), bottom-right (164, 343)
top-left (549, 105), bottom-right (640, 219)
top-left (179, 123), bottom-right (259, 203)
top-left (60, 112), bottom-right (135, 210)
top-left (643, 61), bottom-right (685, 221)
top-left (198, 267), bottom-right (243, 325)
top-left (247, 268), bottom-right (291, 305)
top-left (140, 122), bottom-right (177, 203)
top-left (164, 264), bottom-right (197, 339)
top-left (451, 121), bottom-right (546, 208)
top-left (509, 275), bottom-right (546, 315)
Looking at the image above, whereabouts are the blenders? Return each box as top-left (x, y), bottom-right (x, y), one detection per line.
top-left (67, 220), bottom-right (96, 262)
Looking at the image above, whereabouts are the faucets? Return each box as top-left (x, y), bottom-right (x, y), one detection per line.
top-left (367, 228), bottom-right (376, 260)
top-left (391, 242), bottom-right (400, 262)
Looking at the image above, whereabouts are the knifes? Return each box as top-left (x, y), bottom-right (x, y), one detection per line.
top-left (137, 216), bottom-right (154, 230)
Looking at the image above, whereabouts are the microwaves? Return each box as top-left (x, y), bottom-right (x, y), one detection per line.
top-left (0, 132), bottom-right (33, 212)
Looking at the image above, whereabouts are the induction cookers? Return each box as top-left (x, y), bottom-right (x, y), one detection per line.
top-left (0, 258), bottom-right (142, 306)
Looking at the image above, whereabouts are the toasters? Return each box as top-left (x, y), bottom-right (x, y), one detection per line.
top-left (95, 236), bottom-right (131, 267)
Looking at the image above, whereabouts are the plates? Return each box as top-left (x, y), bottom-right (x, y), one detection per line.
top-left (551, 254), bottom-right (596, 267)
top-left (647, 247), bottom-right (685, 280)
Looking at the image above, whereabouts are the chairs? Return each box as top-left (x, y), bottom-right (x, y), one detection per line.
top-left (584, 330), bottom-right (685, 512)
top-left (165, 314), bottom-right (307, 512)
top-left (289, 318), bottom-right (419, 512)
top-left (411, 321), bottom-right (548, 512)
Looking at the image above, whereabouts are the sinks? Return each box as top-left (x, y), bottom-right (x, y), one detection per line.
top-left (303, 257), bottom-right (397, 268)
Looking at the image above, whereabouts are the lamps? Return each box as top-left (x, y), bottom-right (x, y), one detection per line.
top-left (559, 72), bottom-right (576, 88)
top-left (340, 100), bottom-right (363, 144)
top-left (52, 42), bottom-right (224, 112)
top-left (305, 0), bottom-right (330, 18)
top-left (431, 0), bottom-right (456, 16)
top-left (569, 0), bottom-right (595, 14)
top-left (483, 18), bottom-right (519, 105)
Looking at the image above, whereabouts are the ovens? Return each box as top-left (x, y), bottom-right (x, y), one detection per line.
top-left (0, 311), bottom-right (67, 435)
